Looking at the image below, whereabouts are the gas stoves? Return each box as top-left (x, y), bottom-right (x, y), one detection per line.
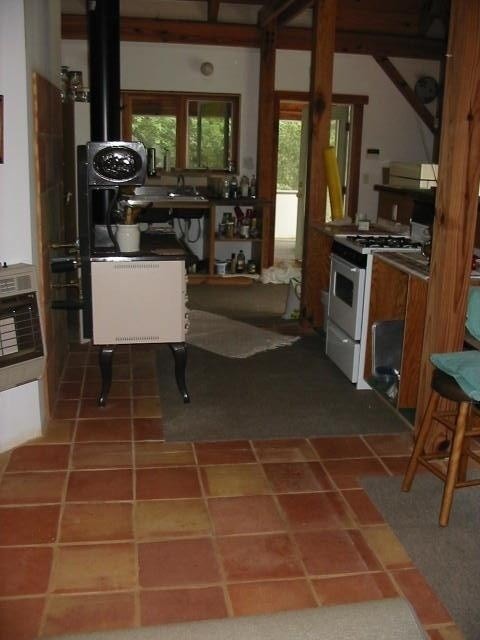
top-left (335, 234), bottom-right (423, 253)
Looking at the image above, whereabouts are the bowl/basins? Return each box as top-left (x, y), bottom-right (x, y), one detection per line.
top-left (375, 367), bottom-right (395, 384)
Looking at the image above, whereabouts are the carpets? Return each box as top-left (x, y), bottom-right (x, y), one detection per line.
top-left (186, 310), bottom-right (301, 359)
top-left (358, 473), bottom-right (479, 639)
top-left (153, 284), bottom-right (412, 444)
top-left (45, 594), bottom-right (431, 639)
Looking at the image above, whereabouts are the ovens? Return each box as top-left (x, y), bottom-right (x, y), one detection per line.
top-left (324, 240), bottom-right (373, 385)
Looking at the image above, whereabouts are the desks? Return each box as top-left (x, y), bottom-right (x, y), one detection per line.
top-left (365, 249), bottom-right (480, 436)
top-left (124, 193), bottom-right (216, 285)
top-left (374, 183), bottom-right (434, 236)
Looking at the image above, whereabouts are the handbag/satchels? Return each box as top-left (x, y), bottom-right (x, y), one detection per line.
top-left (283, 278), bottom-right (302, 320)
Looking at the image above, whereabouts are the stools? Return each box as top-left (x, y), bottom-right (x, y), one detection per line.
top-left (401, 347), bottom-right (480, 528)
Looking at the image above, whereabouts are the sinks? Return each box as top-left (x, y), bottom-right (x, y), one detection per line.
top-left (172, 196), bottom-right (207, 201)
top-left (145, 195), bottom-right (172, 202)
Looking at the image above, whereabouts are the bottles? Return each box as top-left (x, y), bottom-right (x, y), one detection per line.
top-left (113, 223), bottom-right (141, 253)
top-left (189, 259), bottom-right (197, 273)
top-left (164, 150), bottom-right (172, 172)
top-left (223, 161), bottom-right (256, 274)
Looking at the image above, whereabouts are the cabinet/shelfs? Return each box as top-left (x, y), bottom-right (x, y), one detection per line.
top-left (304, 224), bottom-right (340, 332)
top-left (214, 196), bottom-right (266, 285)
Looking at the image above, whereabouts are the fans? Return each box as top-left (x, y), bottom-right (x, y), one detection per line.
top-left (415, 77), bottom-right (439, 104)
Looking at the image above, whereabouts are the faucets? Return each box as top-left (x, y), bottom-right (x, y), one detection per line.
top-left (177, 174), bottom-right (185, 192)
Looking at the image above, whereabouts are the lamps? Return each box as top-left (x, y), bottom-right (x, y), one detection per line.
top-left (201, 61), bottom-right (214, 76)
